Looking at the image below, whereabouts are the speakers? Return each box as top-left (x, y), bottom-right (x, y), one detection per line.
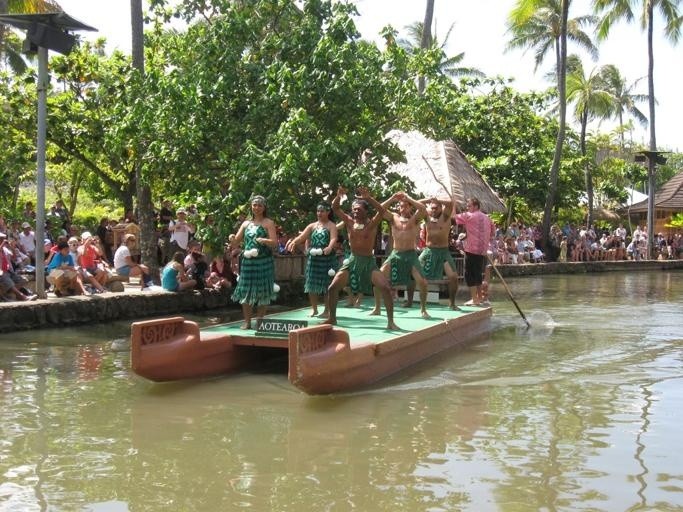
top-left (29, 22), bottom-right (76, 57)
top-left (22, 38), bottom-right (37, 54)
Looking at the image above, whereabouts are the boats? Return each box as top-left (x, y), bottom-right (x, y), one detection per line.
top-left (131, 295), bottom-right (493, 394)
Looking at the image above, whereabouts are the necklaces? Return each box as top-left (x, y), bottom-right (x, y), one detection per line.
top-left (352, 220), bottom-right (369, 233)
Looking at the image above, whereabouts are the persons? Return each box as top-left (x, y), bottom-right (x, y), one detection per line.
top-left (321, 184), bottom-right (400, 331)
top-left (229, 194), bottom-right (278, 330)
top-left (286, 201), bottom-right (335, 320)
top-left (370, 191), bottom-right (434, 320)
top-left (346, 193), bottom-right (545, 283)
top-left (451, 195), bottom-right (496, 306)
top-left (0, 194), bottom-right (150, 304)
top-left (153, 200), bottom-right (237, 293)
top-left (548, 219), bottom-right (683, 262)
top-left (334, 209), bottom-right (368, 309)
top-left (402, 196), bottom-right (463, 310)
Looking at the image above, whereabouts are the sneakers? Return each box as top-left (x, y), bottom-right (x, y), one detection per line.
top-left (26, 294), bottom-right (38, 300)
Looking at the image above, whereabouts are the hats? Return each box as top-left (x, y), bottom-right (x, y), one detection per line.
top-left (176, 207), bottom-right (187, 216)
top-left (82, 232), bottom-right (91, 240)
top-left (22, 222), bottom-right (31, 229)
